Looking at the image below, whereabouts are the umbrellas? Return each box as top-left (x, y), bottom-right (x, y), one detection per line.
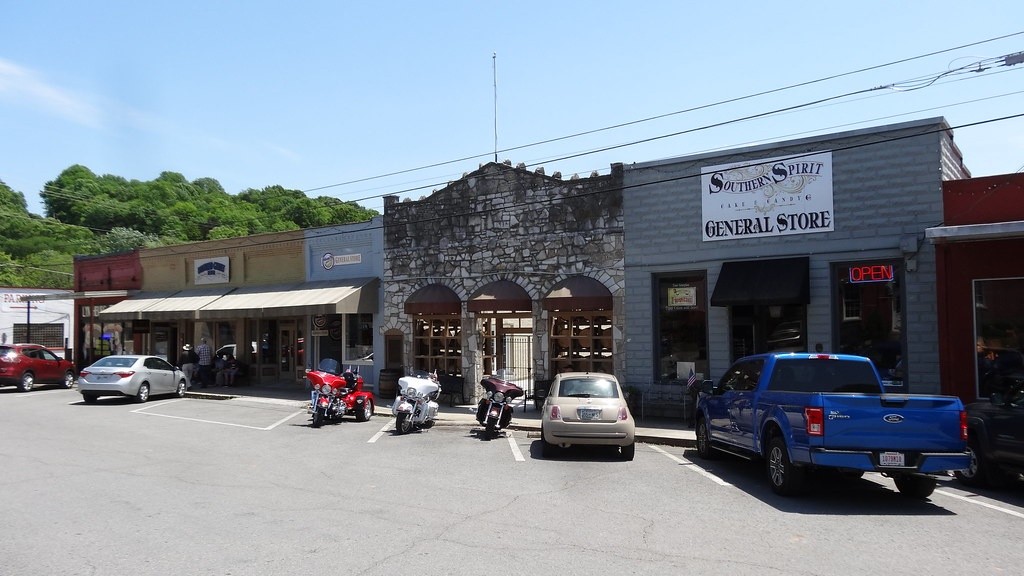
top-left (99, 331), bottom-right (114, 340)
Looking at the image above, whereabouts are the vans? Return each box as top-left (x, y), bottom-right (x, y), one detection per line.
top-left (216, 344), bottom-right (257, 359)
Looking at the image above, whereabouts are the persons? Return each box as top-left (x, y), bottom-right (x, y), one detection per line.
top-left (562, 365), bottom-right (607, 374)
top-left (107, 339), bottom-right (117, 355)
top-left (179, 337), bottom-right (239, 390)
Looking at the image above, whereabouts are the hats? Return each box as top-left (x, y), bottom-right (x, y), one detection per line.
top-left (201, 337), bottom-right (208, 341)
top-left (182, 343), bottom-right (193, 350)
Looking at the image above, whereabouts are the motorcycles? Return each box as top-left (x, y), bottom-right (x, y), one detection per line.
top-left (303, 358), bottom-right (375, 428)
top-left (391, 369), bottom-right (442, 435)
top-left (476, 369), bottom-right (523, 441)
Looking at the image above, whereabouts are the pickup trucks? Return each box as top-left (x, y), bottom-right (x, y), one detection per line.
top-left (693, 352), bottom-right (970, 494)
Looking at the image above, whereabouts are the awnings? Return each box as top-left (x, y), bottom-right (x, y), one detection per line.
top-left (142, 287), bottom-right (238, 321)
top-left (199, 276), bottom-right (380, 321)
top-left (99, 287), bottom-right (182, 322)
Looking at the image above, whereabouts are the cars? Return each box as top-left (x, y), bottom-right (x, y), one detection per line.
top-left (77, 354), bottom-right (189, 403)
top-left (953, 385), bottom-right (1024, 487)
top-left (541, 371), bottom-right (635, 461)
top-left (0, 343), bottom-right (76, 393)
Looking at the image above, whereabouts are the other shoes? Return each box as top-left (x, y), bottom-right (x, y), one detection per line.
top-left (229, 385), bottom-right (232, 388)
top-left (225, 385), bottom-right (228, 387)
top-left (200, 386), bottom-right (207, 388)
top-left (188, 386), bottom-right (192, 390)
